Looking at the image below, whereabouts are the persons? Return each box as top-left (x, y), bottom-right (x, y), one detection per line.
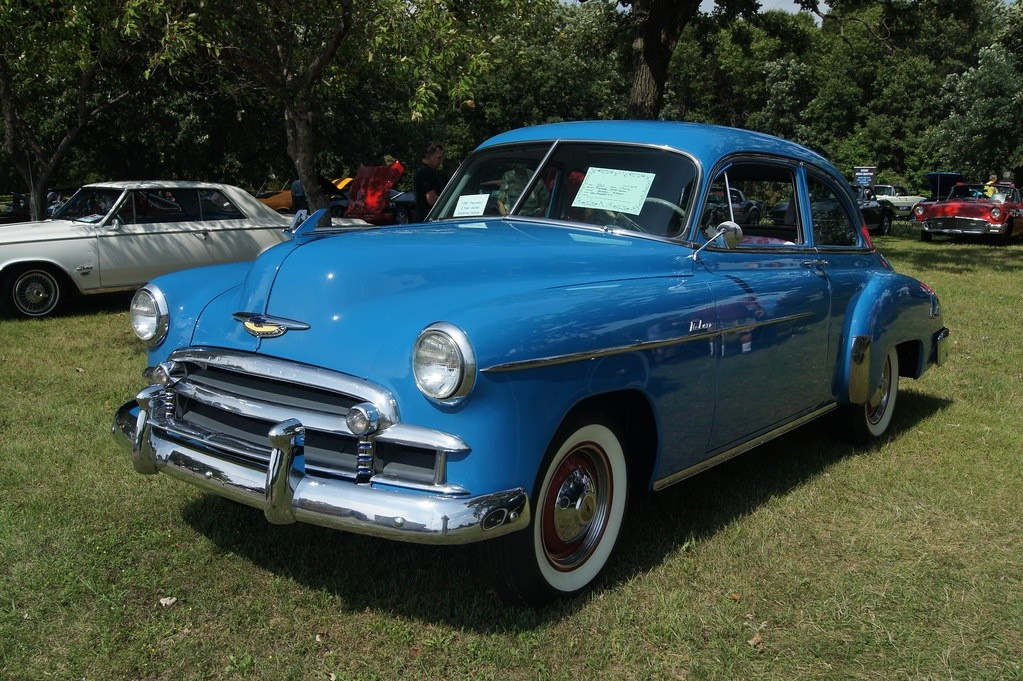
top-left (983, 174), bottom-right (998, 198)
top-left (414, 145), bottom-right (444, 222)
top-left (98, 198), bottom-right (106, 213)
top-left (497, 162), bottom-right (550, 217)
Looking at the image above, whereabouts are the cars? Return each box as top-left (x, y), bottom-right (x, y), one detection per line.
top-left (0, 180), bottom-right (373, 321)
top-left (110, 120), bottom-right (951, 607)
top-left (912, 181), bottom-right (1023, 245)
